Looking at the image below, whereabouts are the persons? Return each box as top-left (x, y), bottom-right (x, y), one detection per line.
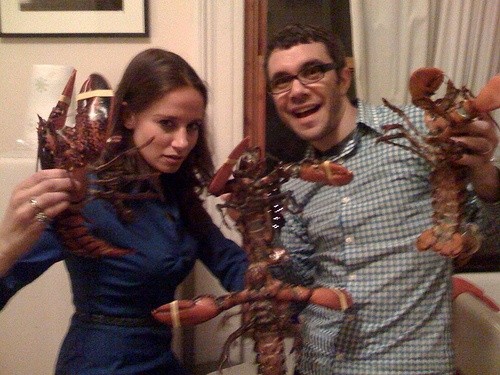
top-left (218, 19), bottom-right (500, 375)
top-left (0, 48), bottom-right (294, 375)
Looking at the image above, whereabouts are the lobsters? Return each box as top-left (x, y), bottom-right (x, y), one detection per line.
top-left (36, 69), bottom-right (162, 258)
top-left (152, 135), bottom-right (354, 374)
top-left (376, 67), bottom-right (500, 265)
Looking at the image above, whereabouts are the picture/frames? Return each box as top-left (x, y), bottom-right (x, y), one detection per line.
top-left (1, 1), bottom-right (150, 38)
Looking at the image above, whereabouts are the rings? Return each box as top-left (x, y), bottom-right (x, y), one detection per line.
top-left (32, 211), bottom-right (55, 224)
top-left (29, 197), bottom-right (44, 212)
top-left (478, 143), bottom-right (494, 155)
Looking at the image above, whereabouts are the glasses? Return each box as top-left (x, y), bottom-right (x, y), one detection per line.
top-left (265, 62), bottom-right (338, 96)
